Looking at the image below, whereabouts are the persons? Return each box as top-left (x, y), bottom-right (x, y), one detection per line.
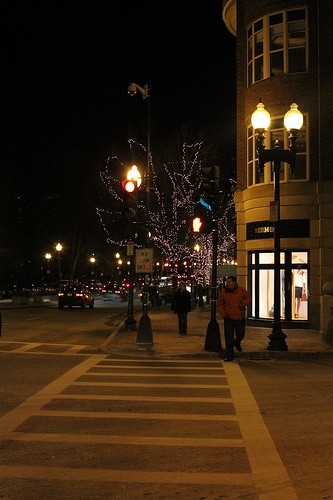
top-left (295, 269), bottom-right (305, 318)
top-left (171, 283), bottom-right (192, 335)
top-left (218, 276), bottom-right (251, 361)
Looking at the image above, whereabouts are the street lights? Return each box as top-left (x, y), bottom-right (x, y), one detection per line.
top-left (119, 164), bottom-right (141, 331)
top-left (45, 253), bottom-right (52, 283)
top-left (251, 96), bottom-right (305, 351)
top-left (56, 242), bottom-right (62, 280)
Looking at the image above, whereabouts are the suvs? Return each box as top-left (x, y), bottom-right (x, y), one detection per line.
top-left (58, 284), bottom-right (95, 310)
top-left (140, 286), bottom-right (160, 302)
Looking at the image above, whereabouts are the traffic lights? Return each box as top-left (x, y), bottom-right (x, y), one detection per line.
top-left (192, 217), bottom-right (203, 235)
top-left (122, 179), bottom-right (137, 194)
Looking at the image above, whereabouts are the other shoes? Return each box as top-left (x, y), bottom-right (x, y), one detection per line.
top-left (234, 341), bottom-right (242, 351)
top-left (224, 357), bottom-right (233, 362)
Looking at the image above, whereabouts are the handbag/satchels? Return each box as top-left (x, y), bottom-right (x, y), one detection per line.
top-left (173, 301), bottom-right (177, 314)
